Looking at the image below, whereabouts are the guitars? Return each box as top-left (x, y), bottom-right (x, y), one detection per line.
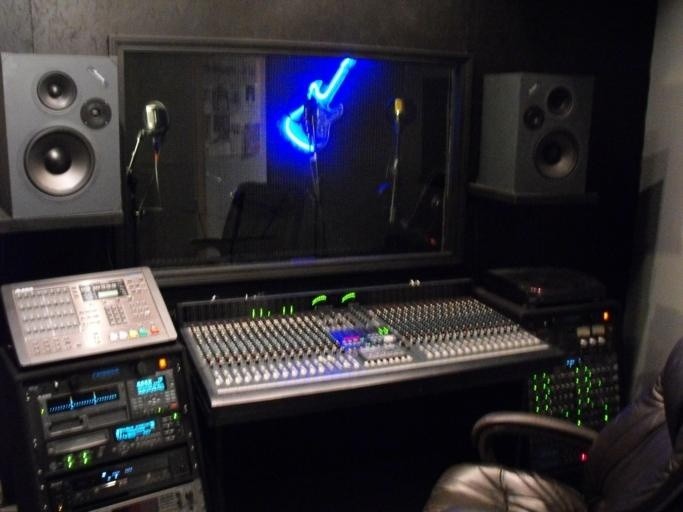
top-left (282, 58), bottom-right (358, 152)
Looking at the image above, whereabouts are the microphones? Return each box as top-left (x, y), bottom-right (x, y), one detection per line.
top-left (394, 98), bottom-right (406, 117)
top-left (145, 100), bottom-right (170, 157)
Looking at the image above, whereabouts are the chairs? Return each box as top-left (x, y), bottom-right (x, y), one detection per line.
top-left (421, 337), bottom-right (683, 512)
top-left (189, 182), bottom-right (305, 262)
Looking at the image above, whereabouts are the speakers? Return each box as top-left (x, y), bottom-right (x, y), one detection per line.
top-left (475, 71), bottom-right (594, 198)
top-left (0, 52), bottom-right (124, 221)
